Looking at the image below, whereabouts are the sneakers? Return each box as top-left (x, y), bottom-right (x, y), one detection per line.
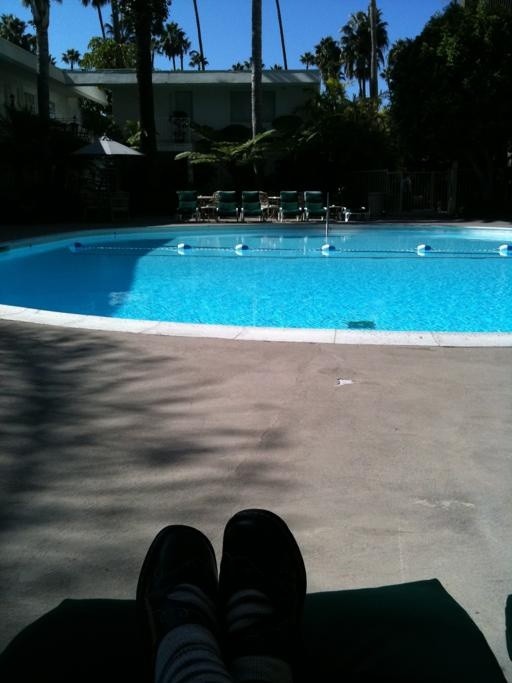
top-left (218, 508), bottom-right (310, 668)
top-left (134, 521), bottom-right (220, 683)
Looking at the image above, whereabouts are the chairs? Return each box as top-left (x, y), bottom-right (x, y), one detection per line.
top-left (177, 190), bottom-right (366, 222)
top-left (1, 578), bottom-right (512, 682)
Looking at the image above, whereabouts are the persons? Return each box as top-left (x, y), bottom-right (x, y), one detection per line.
top-left (136, 509), bottom-right (307, 682)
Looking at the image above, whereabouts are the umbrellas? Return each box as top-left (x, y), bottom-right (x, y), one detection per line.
top-left (71, 132), bottom-right (145, 157)
